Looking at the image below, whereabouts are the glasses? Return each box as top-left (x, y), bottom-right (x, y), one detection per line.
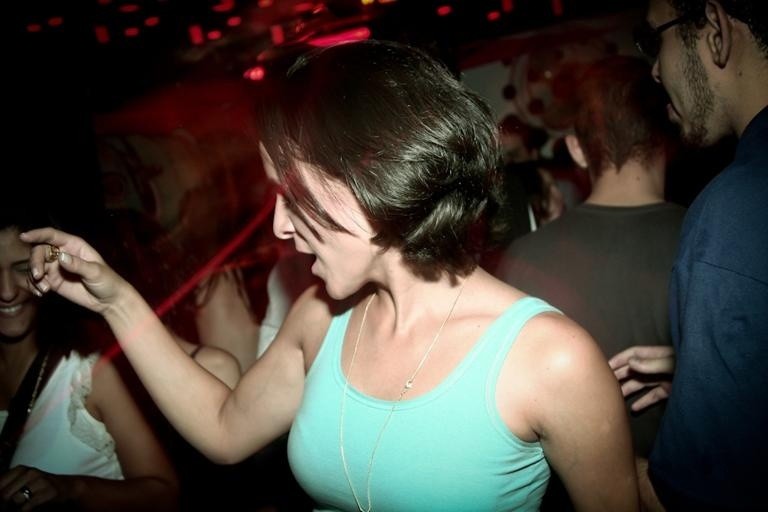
top-left (634, 10), bottom-right (680, 55)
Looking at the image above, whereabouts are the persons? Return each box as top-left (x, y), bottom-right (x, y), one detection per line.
top-left (608, 1), bottom-right (768, 511)
top-left (19, 40), bottom-right (643, 512)
top-left (429, 19), bottom-right (692, 510)
top-left (2, 196), bottom-right (315, 509)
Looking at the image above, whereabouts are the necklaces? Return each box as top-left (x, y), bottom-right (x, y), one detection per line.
top-left (340, 279), bottom-right (463, 512)
